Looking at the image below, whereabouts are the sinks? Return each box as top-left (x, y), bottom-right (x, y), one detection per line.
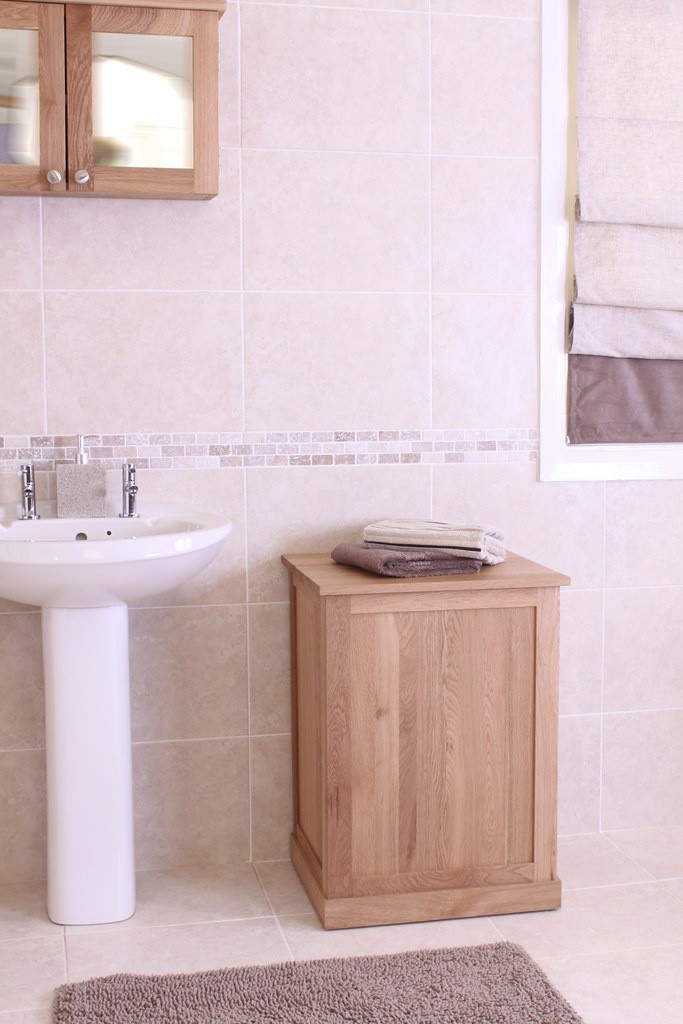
top-left (4, 514), bottom-right (234, 608)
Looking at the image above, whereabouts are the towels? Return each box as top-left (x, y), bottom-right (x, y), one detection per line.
top-left (331, 515), bottom-right (512, 583)
top-left (564, 0), bottom-right (683, 448)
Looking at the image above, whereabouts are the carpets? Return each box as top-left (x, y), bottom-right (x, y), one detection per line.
top-left (53, 941), bottom-right (588, 1024)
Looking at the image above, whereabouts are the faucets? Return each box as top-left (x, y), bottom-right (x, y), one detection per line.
top-left (54, 432), bottom-right (109, 518)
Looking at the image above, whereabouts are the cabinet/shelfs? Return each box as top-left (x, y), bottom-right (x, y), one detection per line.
top-left (0, 0), bottom-right (227, 200)
top-left (280, 549), bottom-right (571, 933)
